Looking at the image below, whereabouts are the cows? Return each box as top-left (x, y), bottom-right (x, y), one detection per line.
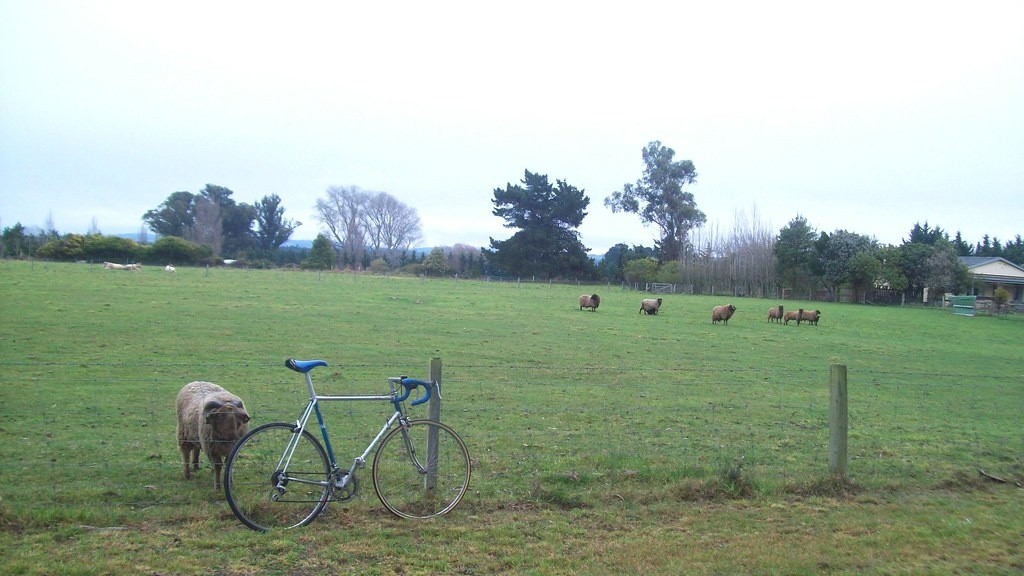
top-left (165, 263), bottom-right (176, 272)
top-left (103, 260), bottom-right (142, 272)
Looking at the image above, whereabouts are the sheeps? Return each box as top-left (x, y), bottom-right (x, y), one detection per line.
top-left (639, 298), bottom-right (662, 315)
top-left (784, 309), bottom-right (820, 326)
top-left (175, 381), bottom-right (250, 489)
top-left (767, 305), bottom-right (783, 323)
top-left (580, 294), bottom-right (600, 312)
top-left (711, 304), bottom-right (736, 325)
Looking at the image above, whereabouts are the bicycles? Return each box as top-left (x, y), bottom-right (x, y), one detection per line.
top-left (223, 359), bottom-right (472, 534)
